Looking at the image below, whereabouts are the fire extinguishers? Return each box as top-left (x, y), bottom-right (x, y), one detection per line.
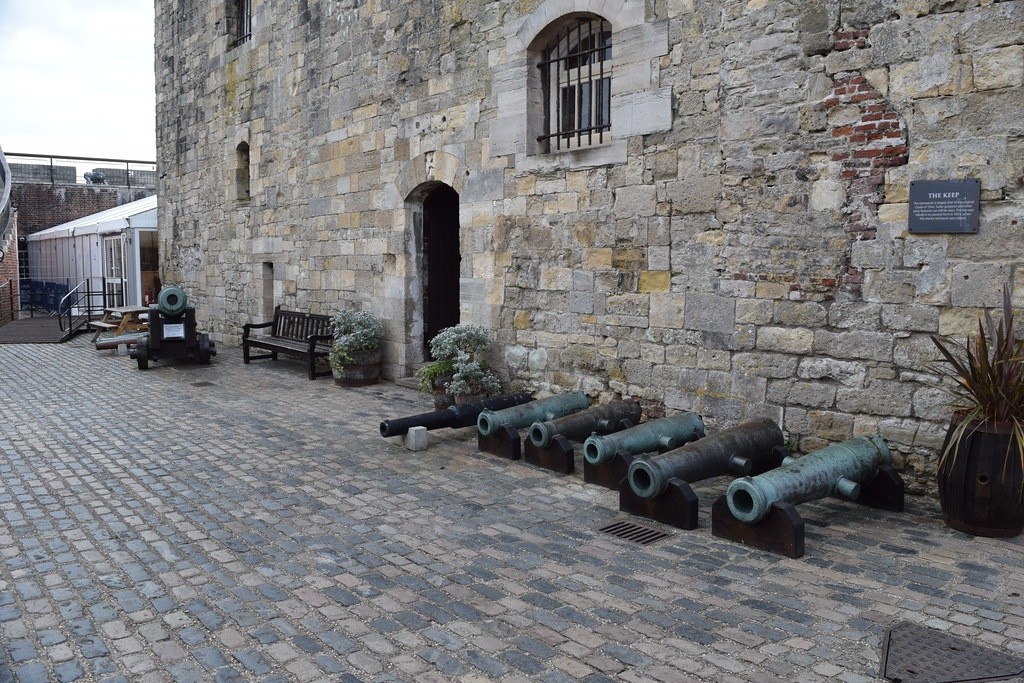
top-left (144, 286), bottom-right (152, 307)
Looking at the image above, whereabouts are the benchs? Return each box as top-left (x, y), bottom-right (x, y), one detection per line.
top-left (242, 305), bottom-right (334, 380)
top-left (89, 321), bottom-right (118, 328)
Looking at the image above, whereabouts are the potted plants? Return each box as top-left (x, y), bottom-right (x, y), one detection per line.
top-left (923, 282), bottom-right (1024, 537)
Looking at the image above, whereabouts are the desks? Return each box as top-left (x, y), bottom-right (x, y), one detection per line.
top-left (91, 306), bottom-right (149, 342)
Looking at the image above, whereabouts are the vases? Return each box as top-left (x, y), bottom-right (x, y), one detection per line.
top-left (430, 375), bottom-right (455, 411)
top-left (332, 349), bottom-right (381, 387)
top-left (454, 393), bottom-right (488, 405)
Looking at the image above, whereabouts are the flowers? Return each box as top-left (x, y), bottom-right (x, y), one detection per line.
top-left (327, 306), bottom-right (385, 378)
top-left (415, 324), bottom-right (501, 404)
